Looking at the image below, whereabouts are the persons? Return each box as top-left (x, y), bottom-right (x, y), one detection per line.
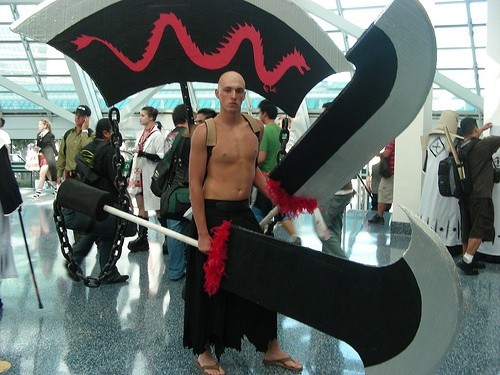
top-left (305, 329), bottom-right (343, 375)
top-left (419, 110), bottom-right (492, 257)
top-left (127, 251), bottom-right (170, 370)
top-left (195, 109), bottom-right (217, 126)
top-left (128, 107), bottom-right (168, 254)
top-left (183, 71), bottom-right (304, 375)
top-left (368, 139), bottom-right (395, 225)
top-left (252, 100), bottom-right (301, 245)
top-left (31, 199), bottom-right (59, 281)
top-left (63, 253), bottom-right (138, 375)
top-left (456, 117), bottom-right (500, 275)
top-left (63, 118), bottom-right (130, 284)
top-left (56, 105), bottom-right (96, 185)
top-left (31, 119), bottom-right (57, 199)
top-left (0, 118), bottom-right (24, 308)
top-left (163, 104), bottom-right (190, 281)
top-left (315, 179), bottom-right (354, 258)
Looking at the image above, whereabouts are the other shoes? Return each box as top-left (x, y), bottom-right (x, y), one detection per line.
top-left (102, 275), bottom-right (129, 284)
top-left (292, 237), bottom-right (302, 245)
top-left (52, 187), bottom-right (57, 196)
top-left (33, 190), bottom-right (42, 198)
top-left (456, 258), bottom-right (479, 275)
top-left (65, 263), bottom-right (80, 282)
top-left (0, 360), bottom-right (11, 373)
top-left (368, 213), bottom-right (384, 225)
top-left (472, 258), bottom-right (487, 269)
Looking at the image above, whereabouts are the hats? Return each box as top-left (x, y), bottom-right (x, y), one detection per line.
top-left (72, 106), bottom-right (91, 117)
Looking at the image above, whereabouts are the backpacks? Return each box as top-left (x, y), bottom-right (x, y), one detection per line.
top-left (159, 114), bottom-right (261, 220)
top-left (438, 137), bottom-right (481, 197)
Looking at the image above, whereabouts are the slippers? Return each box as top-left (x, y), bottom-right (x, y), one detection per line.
top-left (195, 360), bottom-right (226, 375)
top-left (263, 355), bottom-right (303, 371)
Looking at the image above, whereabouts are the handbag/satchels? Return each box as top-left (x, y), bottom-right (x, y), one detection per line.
top-left (150, 150), bottom-right (173, 198)
top-left (60, 208), bottom-right (92, 233)
top-left (378, 157), bottom-right (391, 176)
top-left (24, 143), bottom-right (40, 171)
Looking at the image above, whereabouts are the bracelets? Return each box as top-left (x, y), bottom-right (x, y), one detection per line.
top-left (82, 130), bottom-right (88, 133)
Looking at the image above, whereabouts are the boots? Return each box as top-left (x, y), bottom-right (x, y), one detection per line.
top-left (157, 216), bottom-right (169, 254)
top-left (128, 215), bottom-right (149, 251)
